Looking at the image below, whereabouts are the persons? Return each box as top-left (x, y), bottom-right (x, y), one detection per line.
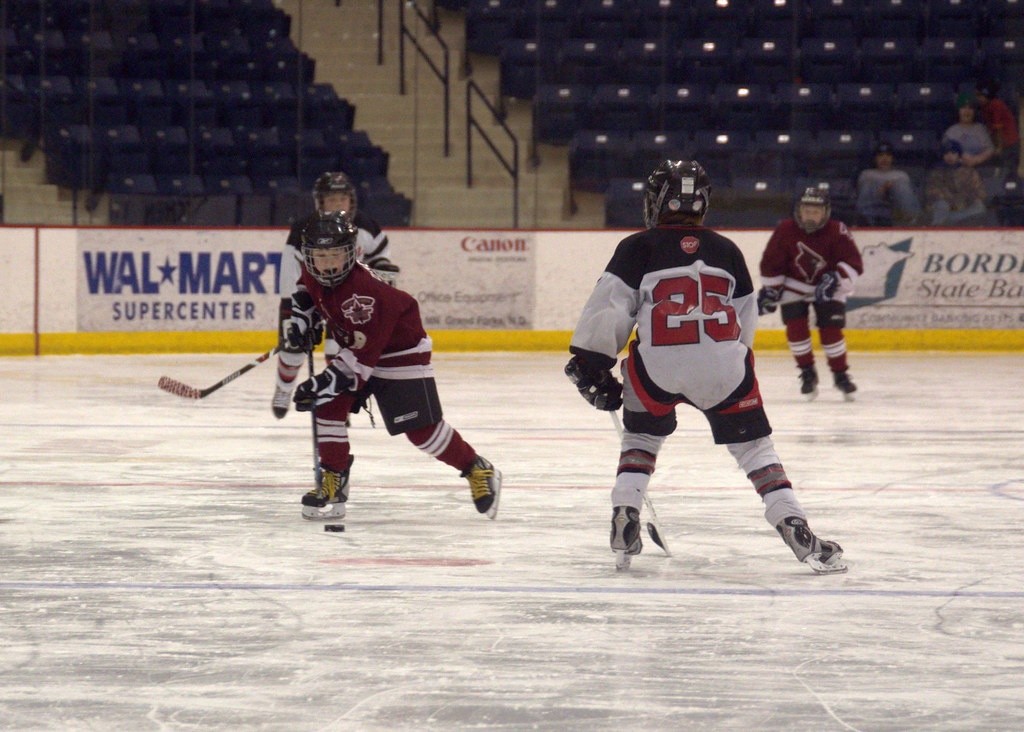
top-left (847, 141), bottom-right (922, 225)
top-left (269, 167), bottom-right (399, 417)
top-left (976, 75), bottom-right (1019, 179)
top-left (940, 92), bottom-right (995, 187)
top-left (563, 156), bottom-right (850, 574)
top-left (756, 184), bottom-right (861, 410)
top-left (285, 214), bottom-right (501, 525)
top-left (923, 141), bottom-right (988, 225)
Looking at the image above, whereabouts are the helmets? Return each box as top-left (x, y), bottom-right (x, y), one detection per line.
top-left (314, 172), bottom-right (358, 221)
top-left (798, 179), bottom-right (831, 206)
top-left (300, 209), bottom-right (359, 286)
top-left (644, 158), bottom-right (712, 223)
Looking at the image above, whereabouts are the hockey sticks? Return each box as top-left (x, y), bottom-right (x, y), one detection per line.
top-left (306, 338), bottom-right (323, 500)
top-left (596, 372), bottom-right (673, 558)
top-left (763, 292), bottom-right (817, 307)
top-left (157, 345), bottom-right (281, 400)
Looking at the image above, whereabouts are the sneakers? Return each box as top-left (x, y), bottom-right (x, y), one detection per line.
top-left (776, 516), bottom-right (848, 575)
top-left (459, 454), bottom-right (502, 519)
top-left (833, 370), bottom-right (857, 402)
top-left (272, 384), bottom-right (292, 419)
top-left (301, 454), bottom-right (354, 522)
top-left (799, 364), bottom-right (818, 402)
top-left (610, 506), bottom-right (643, 570)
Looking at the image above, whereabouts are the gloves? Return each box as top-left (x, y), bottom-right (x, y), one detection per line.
top-left (756, 285), bottom-right (779, 316)
top-left (814, 275), bottom-right (840, 303)
top-left (292, 363), bottom-right (356, 412)
top-left (566, 354), bottom-right (624, 411)
top-left (293, 289), bottom-right (325, 354)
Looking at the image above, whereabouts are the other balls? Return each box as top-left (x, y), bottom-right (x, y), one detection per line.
top-left (324, 524), bottom-right (345, 532)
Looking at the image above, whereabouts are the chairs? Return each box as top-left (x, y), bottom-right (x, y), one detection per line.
top-left (0, 0), bottom-right (1024, 226)
